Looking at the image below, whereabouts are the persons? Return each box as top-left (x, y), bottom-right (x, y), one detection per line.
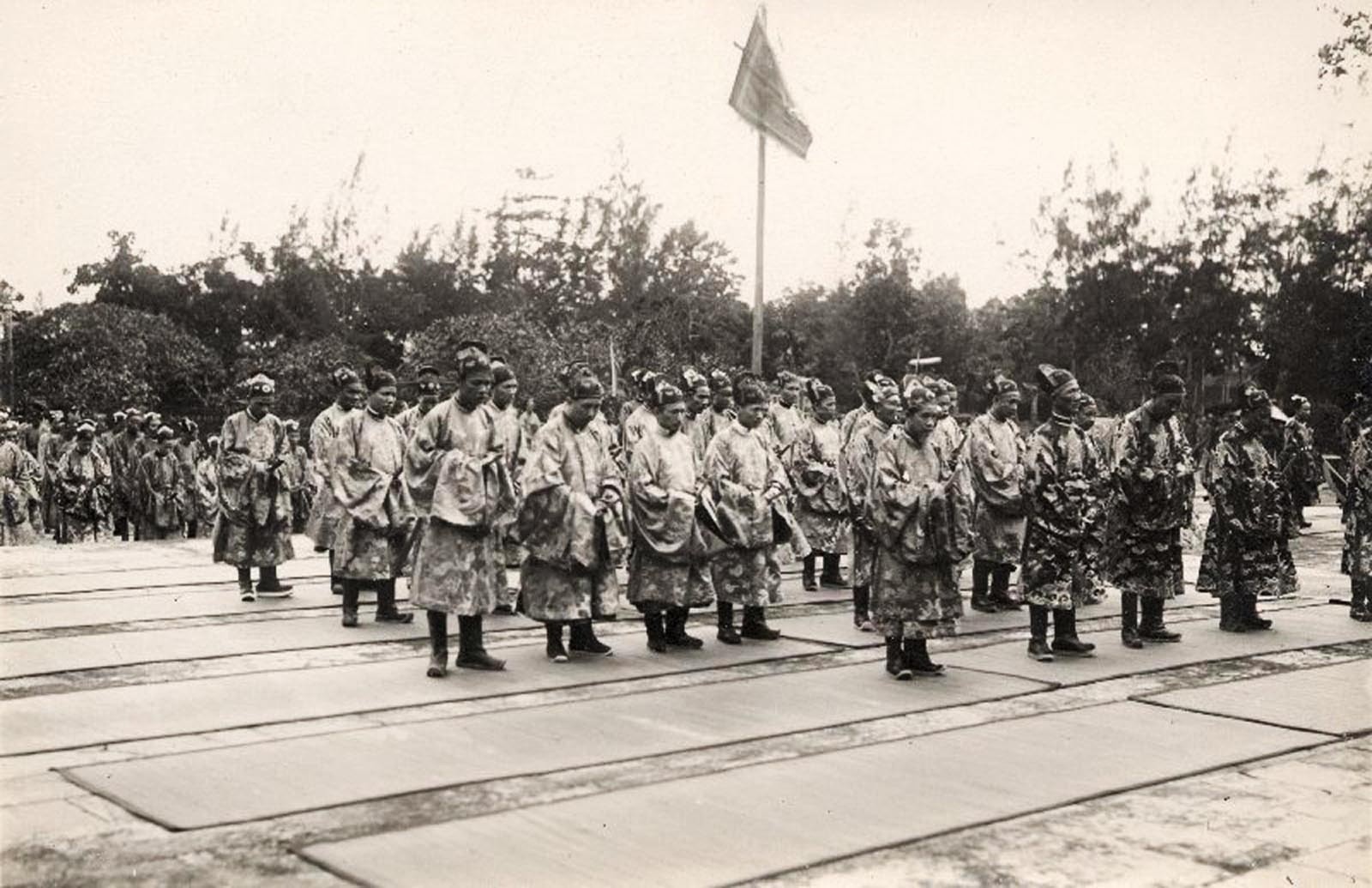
top-left (920, 370), bottom-right (1200, 615)
top-left (1017, 364), bottom-right (1112, 662)
top-left (1339, 393), bottom-right (1372, 622)
top-left (283, 362), bottom-right (443, 627)
top-left (0, 407), bottom-right (220, 544)
top-left (214, 374), bottom-right (301, 601)
top-left (1111, 374), bottom-right (1197, 648)
top-left (403, 347), bottom-right (507, 678)
top-left (485, 355), bottom-right (903, 662)
top-left (871, 374), bottom-right (976, 681)
top-left (1195, 386), bottom-right (1323, 632)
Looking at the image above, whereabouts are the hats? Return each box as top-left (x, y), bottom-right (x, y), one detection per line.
top-left (0, 339), bottom-right (1372, 466)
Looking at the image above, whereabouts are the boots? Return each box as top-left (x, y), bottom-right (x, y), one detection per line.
top-left (545, 624), bottom-right (569, 661)
top-left (666, 609), bottom-right (703, 647)
top-left (821, 553), bottom-right (849, 589)
top-left (1219, 595), bottom-right (1272, 634)
top-left (1028, 607), bottom-right (1055, 660)
top-left (256, 567), bottom-right (294, 597)
top-left (570, 622), bottom-right (613, 655)
top-left (1051, 606), bottom-right (1095, 655)
top-left (1348, 578), bottom-right (1372, 623)
top-left (331, 549), bottom-right (343, 593)
top-left (803, 557), bottom-right (819, 590)
top-left (853, 587), bottom-right (874, 630)
top-left (905, 639), bottom-right (944, 675)
top-left (990, 568), bottom-right (1018, 609)
top-left (886, 636), bottom-right (913, 680)
top-left (376, 583), bottom-right (413, 623)
top-left (743, 606), bottom-right (780, 639)
top-left (1121, 591), bottom-right (1181, 649)
top-left (342, 587), bottom-right (360, 626)
top-left (427, 614), bottom-right (449, 677)
top-left (718, 606), bottom-right (741, 643)
top-left (456, 616), bottom-right (507, 670)
top-left (239, 567), bottom-right (256, 600)
top-left (971, 565), bottom-right (997, 611)
top-left (644, 613), bottom-right (668, 652)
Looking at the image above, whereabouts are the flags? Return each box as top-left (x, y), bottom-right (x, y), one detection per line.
top-left (730, 3), bottom-right (813, 160)
